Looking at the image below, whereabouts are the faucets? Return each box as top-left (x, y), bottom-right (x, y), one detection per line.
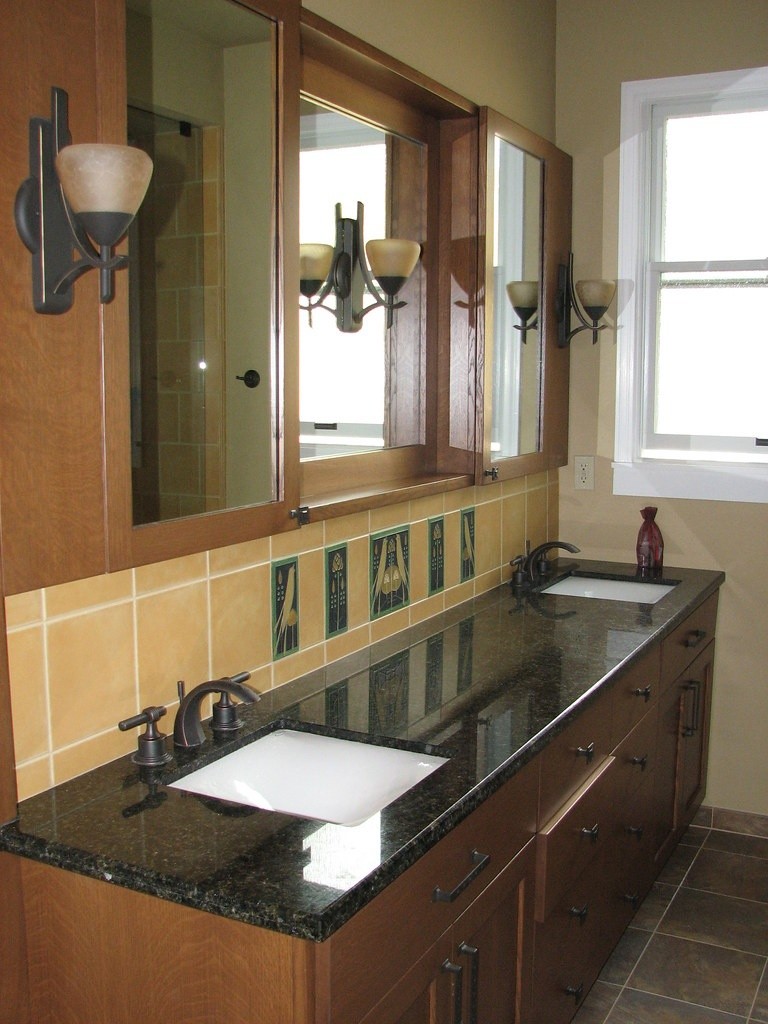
top-left (525, 542), bottom-right (580, 582)
top-left (175, 680), bottom-right (262, 747)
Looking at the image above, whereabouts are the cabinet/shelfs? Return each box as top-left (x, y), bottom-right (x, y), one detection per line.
top-left (13, 584), bottom-right (722, 1024)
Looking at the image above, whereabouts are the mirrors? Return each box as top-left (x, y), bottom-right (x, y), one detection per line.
top-left (92, 0), bottom-right (305, 576)
top-left (476, 102), bottom-right (573, 483)
top-left (298, 52), bottom-right (441, 505)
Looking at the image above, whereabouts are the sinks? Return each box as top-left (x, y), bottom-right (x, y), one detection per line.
top-left (168, 728), bottom-right (449, 825)
top-left (541, 575), bottom-right (677, 603)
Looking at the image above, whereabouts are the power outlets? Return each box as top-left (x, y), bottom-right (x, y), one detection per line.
top-left (574, 456), bottom-right (595, 491)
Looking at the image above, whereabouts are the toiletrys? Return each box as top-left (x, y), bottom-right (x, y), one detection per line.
top-left (636, 506), bottom-right (664, 568)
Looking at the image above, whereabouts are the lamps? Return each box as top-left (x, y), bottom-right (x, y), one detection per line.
top-left (504, 275), bottom-right (538, 344)
top-left (558, 251), bottom-right (618, 350)
top-left (300, 201), bottom-right (343, 335)
top-left (337, 199), bottom-right (422, 333)
top-left (12, 85), bottom-right (154, 316)
